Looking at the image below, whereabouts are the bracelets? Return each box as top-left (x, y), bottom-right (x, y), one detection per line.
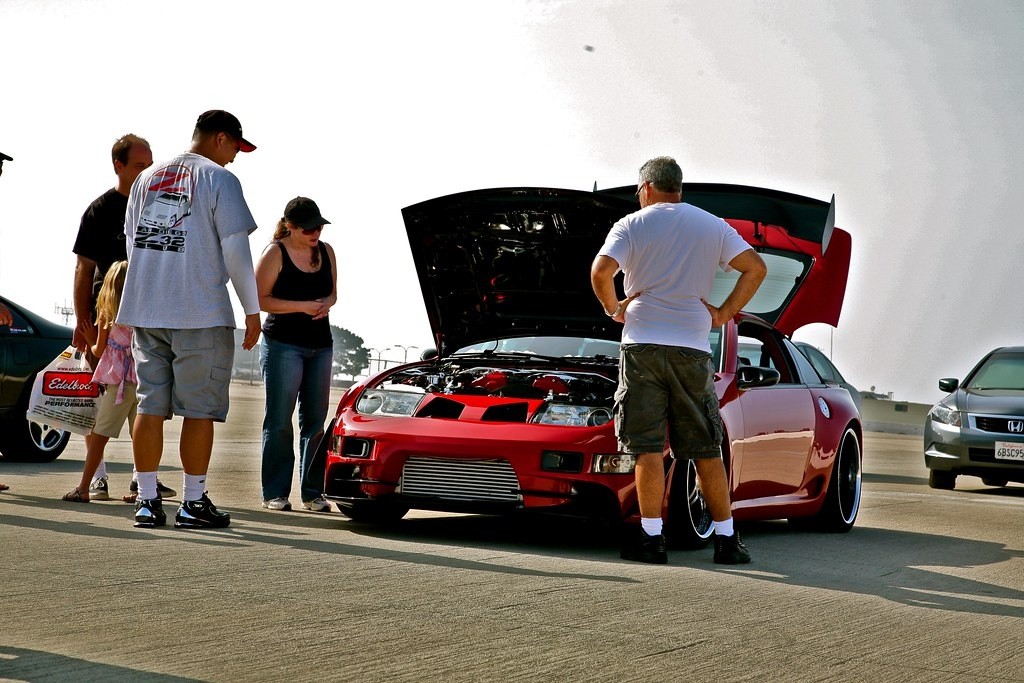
top-left (605, 303), bottom-right (621, 318)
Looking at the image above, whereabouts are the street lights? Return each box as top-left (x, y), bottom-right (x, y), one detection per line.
top-left (370, 348), bottom-right (390, 372)
top-left (395, 345), bottom-right (419, 364)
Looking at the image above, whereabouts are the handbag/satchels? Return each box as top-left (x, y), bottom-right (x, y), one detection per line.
top-left (27, 344), bottom-right (98, 436)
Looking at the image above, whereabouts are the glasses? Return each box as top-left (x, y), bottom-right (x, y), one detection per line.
top-left (635, 180), bottom-right (652, 200)
top-left (300, 227), bottom-right (323, 234)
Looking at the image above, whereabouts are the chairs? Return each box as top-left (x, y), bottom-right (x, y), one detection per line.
top-left (582, 342), bottom-right (620, 359)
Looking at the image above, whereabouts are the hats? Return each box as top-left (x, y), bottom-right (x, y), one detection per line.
top-left (285, 196), bottom-right (332, 230)
top-left (196, 109), bottom-right (257, 153)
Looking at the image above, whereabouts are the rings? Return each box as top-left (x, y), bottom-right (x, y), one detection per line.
top-left (0, 320), bottom-right (3, 322)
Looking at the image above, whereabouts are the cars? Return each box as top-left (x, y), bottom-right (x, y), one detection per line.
top-left (300, 182), bottom-right (863, 552)
top-left (738, 342), bottom-right (863, 417)
top-left (924, 346), bottom-right (1024, 489)
top-left (0, 296), bottom-right (81, 462)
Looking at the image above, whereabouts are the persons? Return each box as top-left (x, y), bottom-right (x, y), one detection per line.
top-left (591, 156), bottom-right (767, 564)
top-left (72, 135), bottom-right (177, 503)
top-left (114, 110), bottom-right (261, 528)
top-left (0, 152), bottom-right (13, 493)
top-left (61, 260), bottom-right (137, 503)
top-left (254, 196), bottom-right (338, 513)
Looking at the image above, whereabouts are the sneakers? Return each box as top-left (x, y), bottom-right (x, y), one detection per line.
top-left (129, 478), bottom-right (177, 497)
top-left (173, 491), bottom-right (231, 529)
top-left (620, 526), bottom-right (668, 565)
top-left (89, 478), bottom-right (110, 500)
top-left (301, 496), bottom-right (332, 512)
top-left (134, 488), bottom-right (167, 528)
top-left (713, 531), bottom-right (751, 565)
top-left (261, 496), bottom-right (293, 510)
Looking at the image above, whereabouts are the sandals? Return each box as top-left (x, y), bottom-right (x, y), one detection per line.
top-left (123, 488), bottom-right (164, 502)
top-left (60, 486), bottom-right (90, 504)
